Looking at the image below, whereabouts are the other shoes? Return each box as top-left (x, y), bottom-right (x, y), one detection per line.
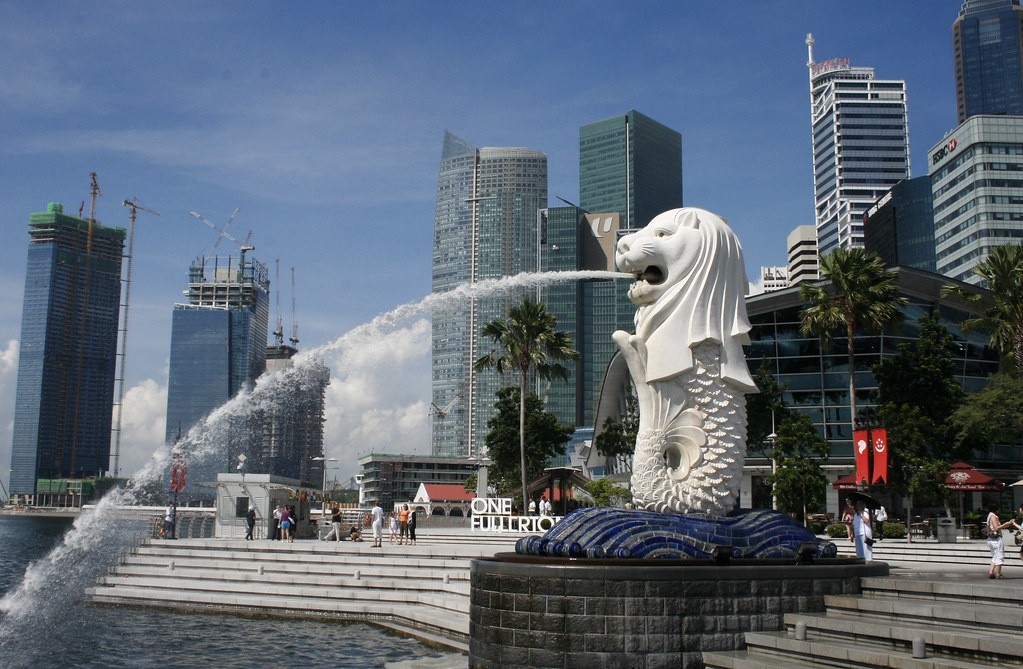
top-left (988, 572), bottom-right (995, 578)
top-left (324, 537), bottom-right (328, 542)
top-left (847, 537), bottom-right (852, 540)
top-left (245, 537), bottom-right (249, 540)
top-left (398, 543), bottom-right (403, 545)
top-left (372, 544), bottom-right (381, 547)
top-left (996, 574), bottom-right (1005, 578)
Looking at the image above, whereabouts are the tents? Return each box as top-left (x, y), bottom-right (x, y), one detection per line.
top-left (936, 461), bottom-right (1003, 527)
top-left (1008, 479), bottom-right (1023, 488)
top-left (833, 473), bottom-right (881, 490)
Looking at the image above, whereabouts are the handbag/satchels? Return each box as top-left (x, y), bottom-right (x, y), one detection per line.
top-left (884, 512), bottom-right (888, 520)
top-left (288, 517), bottom-right (294, 524)
top-left (982, 515), bottom-right (998, 537)
top-left (864, 537), bottom-right (876, 547)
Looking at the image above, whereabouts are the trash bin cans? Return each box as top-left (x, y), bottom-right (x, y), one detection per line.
top-left (937, 517), bottom-right (957, 542)
top-left (320, 521), bottom-right (335, 541)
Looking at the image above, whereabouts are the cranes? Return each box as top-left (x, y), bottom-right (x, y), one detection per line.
top-left (113, 197), bottom-right (160, 476)
top-left (69, 167), bottom-right (101, 478)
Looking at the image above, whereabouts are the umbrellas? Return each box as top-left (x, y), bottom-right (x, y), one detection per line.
top-left (844, 490), bottom-right (883, 508)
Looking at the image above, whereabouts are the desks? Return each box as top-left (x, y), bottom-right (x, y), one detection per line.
top-left (910, 520), bottom-right (990, 539)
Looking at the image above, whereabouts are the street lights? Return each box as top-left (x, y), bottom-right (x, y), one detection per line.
top-left (312, 457), bottom-right (339, 541)
top-left (767, 432), bottom-right (778, 511)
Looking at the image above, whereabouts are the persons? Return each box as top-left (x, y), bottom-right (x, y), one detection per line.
top-left (323, 503), bottom-right (342, 541)
top-left (397, 503), bottom-right (417, 545)
top-left (388, 510), bottom-right (397, 544)
top-left (271, 504), bottom-right (296, 542)
top-left (874, 504), bottom-right (888, 540)
top-left (985, 503), bottom-right (1012, 579)
top-left (156, 501), bottom-right (175, 539)
top-left (350, 526), bottom-right (364, 542)
top-left (244, 504), bottom-right (256, 540)
top-left (851, 499), bottom-right (873, 561)
top-left (842, 497), bottom-right (855, 542)
top-left (527, 498), bottom-right (536, 516)
top-left (539, 496), bottom-right (553, 516)
top-left (1012, 503), bottom-right (1023, 562)
top-left (371, 499), bottom-right (384, 547)
